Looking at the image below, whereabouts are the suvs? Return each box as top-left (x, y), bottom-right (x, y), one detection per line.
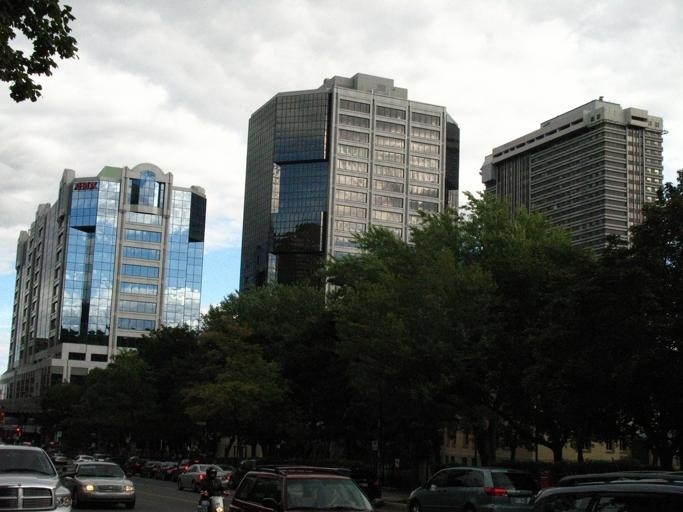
top-left (0, 444), bottom-right (74, 512)
top-left (230, 466), bottom-right (374, 512)
top-left (528, 467), bottom-right (683, 512)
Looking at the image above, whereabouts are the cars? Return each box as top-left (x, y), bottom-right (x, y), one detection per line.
top-left (50, 453), bottom-right (68, 464)
top-left (176, 463), bottom-right (231, 492)
top-left (75, 453), bottom-right (195, 481)
top-left (404, 465), bottom-right (539, 512)
top-left (64, 462), bottom-right (137, 510)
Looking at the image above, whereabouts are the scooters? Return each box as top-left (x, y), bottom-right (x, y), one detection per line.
top-left (200, 491), bottom-right (229, 512)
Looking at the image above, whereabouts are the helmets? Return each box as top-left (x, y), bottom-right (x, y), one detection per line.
top-left (206, 466), bottom-right (218, 478)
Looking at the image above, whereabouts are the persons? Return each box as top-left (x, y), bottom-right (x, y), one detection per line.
top-left (194, 466), bottom-right (231, 512)
